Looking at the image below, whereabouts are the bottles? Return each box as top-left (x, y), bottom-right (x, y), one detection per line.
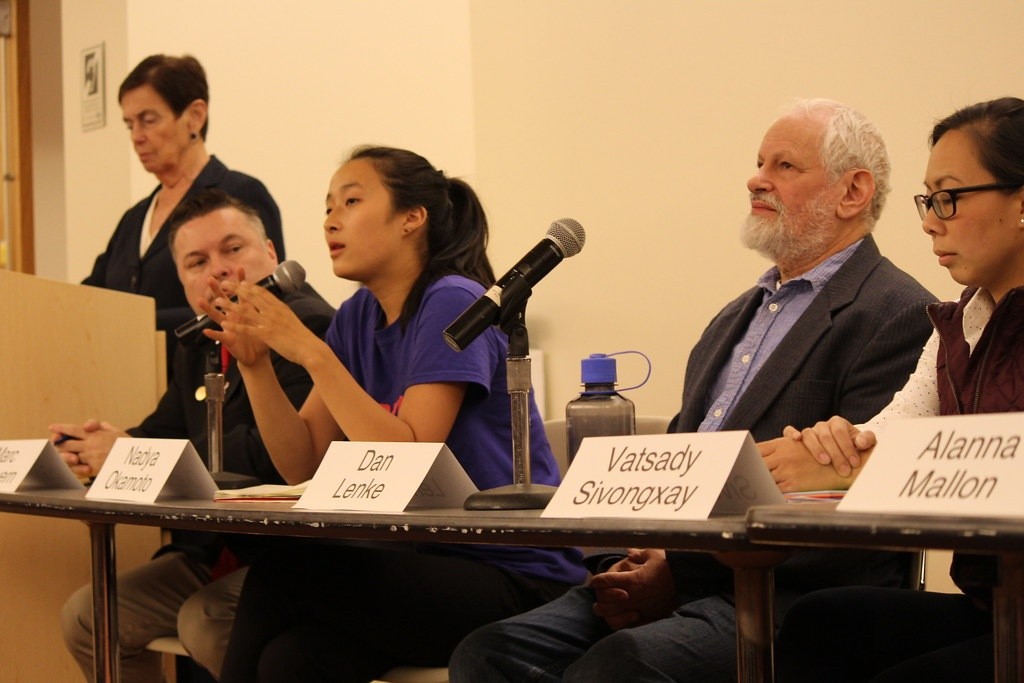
top-left (565, 350), bottom-right (652, 473)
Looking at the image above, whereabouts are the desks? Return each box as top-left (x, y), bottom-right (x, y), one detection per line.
top-left (0, 491), bottom-right (755, 683)
top-left (745, 505), bottom-right (1024, 683)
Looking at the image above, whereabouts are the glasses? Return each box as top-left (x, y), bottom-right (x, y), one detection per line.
top-left (914, 183), bottom-right (1006, 221)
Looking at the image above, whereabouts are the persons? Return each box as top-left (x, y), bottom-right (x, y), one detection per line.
top-left (753, 97), bottom-right (1023, 683)
top-left (447, 96), bottom-right (943, 683)
top-left (47, 185), bottom-right (338, 683)
top-left (196, 146), bottom-right (590, 683)
top-left (73, 46), bottom-right (285, 388)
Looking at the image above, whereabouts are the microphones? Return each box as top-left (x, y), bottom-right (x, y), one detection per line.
top-left (175, 261), bottom-right (306, 343)
top-left (443, 218), bottom-right (586, 353)
